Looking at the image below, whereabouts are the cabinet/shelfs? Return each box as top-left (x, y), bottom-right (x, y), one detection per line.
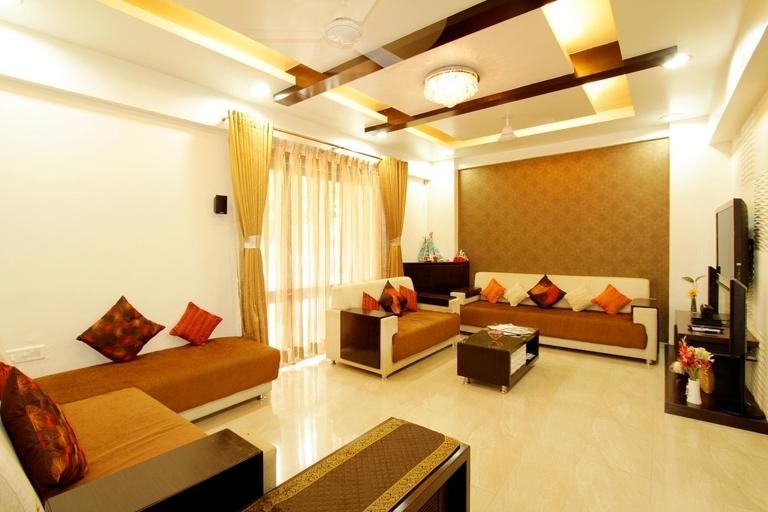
top-left (403, 261), bottom-right (470, 296)
top-left (661, 306), bottom-right (763, 435)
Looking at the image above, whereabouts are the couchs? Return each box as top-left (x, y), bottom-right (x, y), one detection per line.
top-left (448, 270), bottom-right (660, 368)
top-left (323, 274), bottom-right (462, 380)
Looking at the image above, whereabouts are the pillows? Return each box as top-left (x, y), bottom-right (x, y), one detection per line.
top-left (378, 279), bottom-right (408, 317)
top-left (397, 284), bottom-right (420, 313)
top-left (481, 278), bottom-right (505, 304)
top-left (73, 294), bottom-right (167, 363)
top-left (590, 283), bottom-right (632, 317)
top-left (502, 284), bottom-right (530, 308)
top-left (0, 361), bottom-right (90, 490)
top-left (563, 285), bottom-right (594, 313)
top-left (526, 271), bottom-right (567, 310)
top-left (361, 290), bottom-right (380, 311)
top-left (167, 301), bottom-right (224, 346)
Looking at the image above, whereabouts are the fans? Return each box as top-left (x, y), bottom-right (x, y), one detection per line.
top-left (256, 0), bottom-right (406, 74)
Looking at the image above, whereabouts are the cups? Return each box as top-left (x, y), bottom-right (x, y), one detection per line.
top-left (669, 361), bottom-right (682, 374)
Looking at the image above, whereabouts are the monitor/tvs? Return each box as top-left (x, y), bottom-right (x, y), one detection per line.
top-left (714, 198), bottom-right (754, 291)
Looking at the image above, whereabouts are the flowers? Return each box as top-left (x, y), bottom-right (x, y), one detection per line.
top-left (676, 334), bottom-right (716, 381)
top-left (682, 273), bottom-right (706, 298)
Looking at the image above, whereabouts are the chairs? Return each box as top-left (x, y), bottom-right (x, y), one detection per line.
top-left (0, 386), bottom-right (277, 512)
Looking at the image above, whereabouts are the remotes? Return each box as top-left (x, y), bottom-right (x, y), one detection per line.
top-left (691, 326), bottom-right (721, 334)
top-left (687, 324), bottom-right (724, 331)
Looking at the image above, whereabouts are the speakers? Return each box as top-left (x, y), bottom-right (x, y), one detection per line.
top-left (215, 194), bottom-right (227, 214)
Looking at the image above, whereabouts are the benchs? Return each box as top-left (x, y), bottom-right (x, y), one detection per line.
top-left (30, 334), bottom-right (282, 424)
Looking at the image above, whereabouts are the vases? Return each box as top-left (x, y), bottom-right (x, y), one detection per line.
top-left (683, 376), bottom-right (704, 406)
top-left (689, 298), bottom-right (699, 311)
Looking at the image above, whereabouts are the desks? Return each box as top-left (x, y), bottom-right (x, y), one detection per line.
top-left (239, 415), bottom-right (476, 512)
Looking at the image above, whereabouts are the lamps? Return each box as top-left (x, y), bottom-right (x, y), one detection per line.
top-left (497, 115), bottom-right (517, 141)
top-left (422, 63), bottom-right (479, 110)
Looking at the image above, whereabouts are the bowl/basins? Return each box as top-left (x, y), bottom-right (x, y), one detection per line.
top-left (488, 330), bottom-right (504, 341)
top-left (488, 339), bottom-right (503, 350)
